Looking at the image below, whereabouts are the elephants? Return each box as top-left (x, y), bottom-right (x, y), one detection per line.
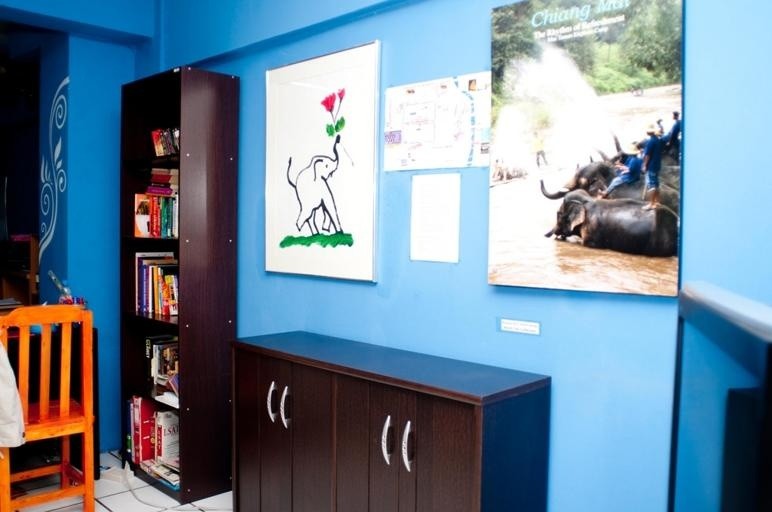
top-left (538, 122), bottom-right (683, 258)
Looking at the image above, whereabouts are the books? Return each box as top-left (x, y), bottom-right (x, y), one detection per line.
top-left (127, 125), bottom-right (180, 494)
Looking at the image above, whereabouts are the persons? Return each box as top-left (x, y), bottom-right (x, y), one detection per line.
top-left (597, 111), bottom-right (681, 199)
top-left (531, 132), bottom-right (550, 169)
top-left (640, 122), bottom-right (663, 211)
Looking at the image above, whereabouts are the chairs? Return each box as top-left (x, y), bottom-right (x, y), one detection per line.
top-left (0, 303), bottom-right (95, 512)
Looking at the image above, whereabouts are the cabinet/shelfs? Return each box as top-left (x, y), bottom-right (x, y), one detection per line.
top-left (233, 342), bottom-right (336, 512)
top-left (118, 67), bottom-right (239, 506)
top-left (334, 374), bottom-right (478, 512)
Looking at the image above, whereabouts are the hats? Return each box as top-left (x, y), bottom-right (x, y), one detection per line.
top-left (620, 143), bottom-right (640, 155)
top-left (644, 122), bottom-right (658, 131)
